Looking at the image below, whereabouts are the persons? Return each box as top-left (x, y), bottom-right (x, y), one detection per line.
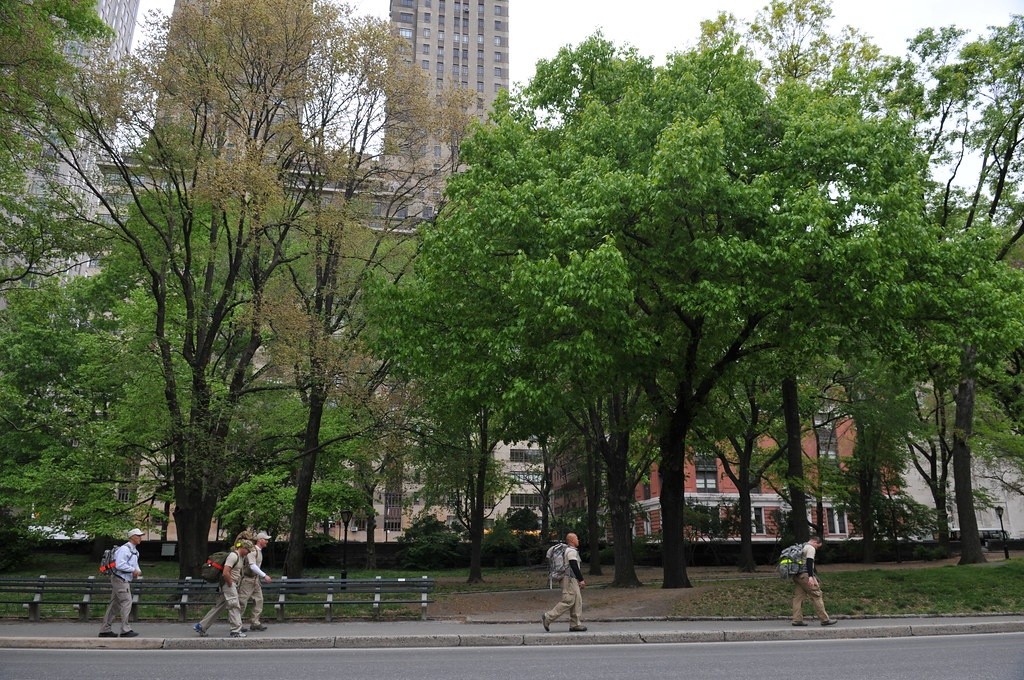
top-left (194, 540), bottom-right (253, 638)
top-left (98, 528), bottom-right (145, 638)
top-left (237, 533), bottom-right (272, 633)
top-left (790, 536), bottom-right (838, 626)
top-left (542, 533), bottom-right (588, 631)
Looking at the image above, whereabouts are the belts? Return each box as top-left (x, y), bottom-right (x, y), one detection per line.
top-left (112, 572), bottom-right (131, 582)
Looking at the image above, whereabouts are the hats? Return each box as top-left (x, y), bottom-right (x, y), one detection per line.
top-left (127, 528), bottom-right (145, 539)
top-left (257, 533), bottom-right (270, 539)
top-left (241, 539), bottom-right (255, 552)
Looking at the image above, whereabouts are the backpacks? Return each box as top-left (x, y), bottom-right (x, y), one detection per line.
top-left (776, 542), bottom-right (815, 578)
top-left (100, 543), bottom-right (132, 575)
top-left (201, 550), bottom-right (239, 583)
top-left (546, 543), bottom-right (578, 582)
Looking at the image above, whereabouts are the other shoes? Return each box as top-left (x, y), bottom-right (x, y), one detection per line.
top-left (230, 632), bottom-right (246, 638)
top-left (120, 630), bottom-right (139, 637)
top-left (542, 613), bottom-right (550, 632)
top-left (250, 624), bottom-right (267, 631)
top-left (241, 628), bottom-right (247, 633)
top-left (792, 621), bottom-right (808, 626)
top-left (569, 625), bottom-right (587, 632)
top-left (821, 620), bottom-right (837, 626)
top-left (193, 623), bottom-right (208, 637)
top-left (99, 631), bottom-right (118, 637)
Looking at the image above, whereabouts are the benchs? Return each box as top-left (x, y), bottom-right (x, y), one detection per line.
top-left (0, 575), bottom-right (438, 621)
